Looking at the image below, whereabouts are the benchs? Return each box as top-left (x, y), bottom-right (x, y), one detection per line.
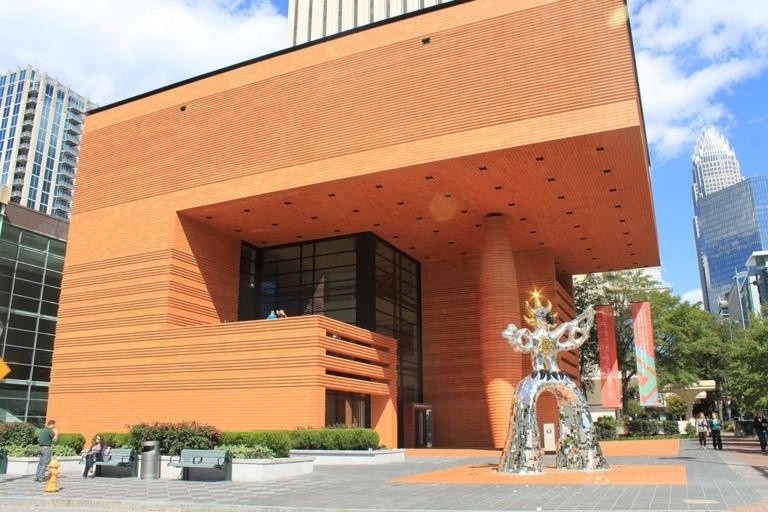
top-left (168, 449), bottom-right (233, 481)
top-left (78, 447), bottom-right (137, 479)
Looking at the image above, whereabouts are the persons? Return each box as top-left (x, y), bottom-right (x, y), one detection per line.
top-left (82, 434), bottom-right (113, 479)
top-left (695, 411), bottom-right (708, 450)
top-left (754, 410), bottom-right (768, 453)
top-left (33, 420), bottom-right (58, 481)
top-left (267, 309), bottom-right (277, 319)
top-left (276, 309), bottom-right (287, 318)
top-left (710, 411), bottom-right (723, 450)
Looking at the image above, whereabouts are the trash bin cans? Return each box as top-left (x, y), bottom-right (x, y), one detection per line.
top-left (141, 441), bottom-right (160, 480)
